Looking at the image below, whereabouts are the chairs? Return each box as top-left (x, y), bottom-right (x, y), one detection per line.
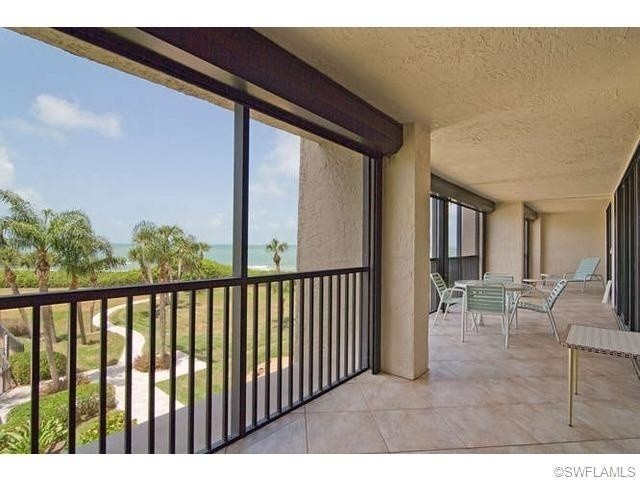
top-left (559, 323), bottom-right (639, 427)
top-left (564, 256), bottom-right (606, 293)
top-left (429, 272), bottom-right (566, 347)
